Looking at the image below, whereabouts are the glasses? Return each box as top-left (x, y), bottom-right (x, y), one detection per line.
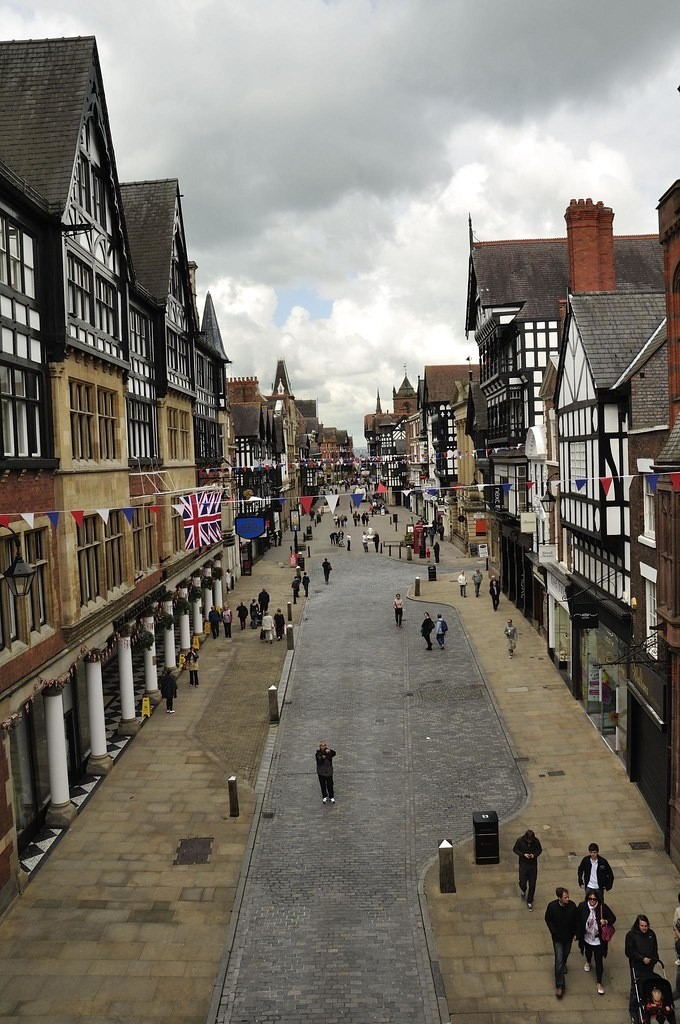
top-left (588, 898), bottom-right (597, 901)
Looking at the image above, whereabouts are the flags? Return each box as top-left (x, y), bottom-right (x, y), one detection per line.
top-left (215, 491), bottom-right (223, 540)
top-left (179, 491), bottom-right (210, 550)
top-left (206, 491), bottom-right (219, 544)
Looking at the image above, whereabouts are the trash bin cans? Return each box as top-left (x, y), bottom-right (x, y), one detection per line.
top-left (316, 514), bottom-right (321, 522)
top-left (419, 545), bottom-right (426, 558)
top-left (393, 513), bottom-right (397, 523)
top-left (473, 811), bottom-right (499, 865)
top-left (427, 565), bottom-right (437, 582)
top-left (307, 525), bottom-right (312, 534)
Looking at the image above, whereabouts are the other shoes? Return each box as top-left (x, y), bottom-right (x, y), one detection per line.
top-left (170, 711), bottom-right (175, 714)
top-left (442, 645), bottom-right (445, 649)
top-left (556, 988), bottom-right (563, 996)
top-left (520, 890), bottom-right (526, 901)
top-left (426, 643), bottom-right (433, 650)
top-left (673, 991), bottom-right (680, 1001)
top-left (564, 965), bottom-right (568, 974)
top-left (584, 963), bottom-right (590, 972)
top-left (597, 988), bottom-right (604, 993)
top-left (277, 636), bottom-right (280, 641)
top-left (527, 903), bottom-right (533, 912)
top-left (331, 798), bottom-right (336, 803)
top-left (167, 710), bottom-right (169, 712)
top-left (322, 797), bottom-right (328, 803)
top-left (674, 960), bottom-right (680, 966)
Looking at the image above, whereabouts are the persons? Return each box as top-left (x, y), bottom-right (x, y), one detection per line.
top-left (671, 892), bottom-right (680, 1000)
top-left (315, 741), bottom-right (337, 805)
top-left (273, 475), bottom-right (445, 597)
top-left (157, 667), bottom-right (178, 714)
top-left (185, 647), bottom-right (199, 688)
top-left (420, 612), bottom-right (449, 651)
top-left (504, 619), bottom-right (517, 659)
top-left (208, 588), bottom-right (285, 645)
top-left (512, 830), bottom-right (542, 912)
top-left (646, 987), bottom-right (670, 1024)
top-left (578, 843), bottom-right (614, 904)
top-left (393, 594), bottom-right (404, 628)
top-left (544, 887), bottom-right (577, 999)
top-left (578, 889), bottom-right (616, 995)
top-left (625, 914), bottom-right (660, 1024)
top-left (457, 568), bottom-right (502, 612)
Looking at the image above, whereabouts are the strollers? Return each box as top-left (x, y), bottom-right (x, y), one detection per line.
top-left (630, 958), bottom-right (677, 1024)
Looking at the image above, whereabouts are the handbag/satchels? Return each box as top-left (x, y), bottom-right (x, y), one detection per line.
top-left (328, 565), bottom-right (332, 570)
top-left (601, 905), bottom-right (615, 942)
top-left (430, 621), bottom-right (435, 630)
top-left (260, 629), bottom-right (266, 640)
top-left (283, 625), bottom-right (287, 635)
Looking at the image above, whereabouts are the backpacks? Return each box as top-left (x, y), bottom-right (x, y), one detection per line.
top-left (436, 619), bottom-right (448, 632)
